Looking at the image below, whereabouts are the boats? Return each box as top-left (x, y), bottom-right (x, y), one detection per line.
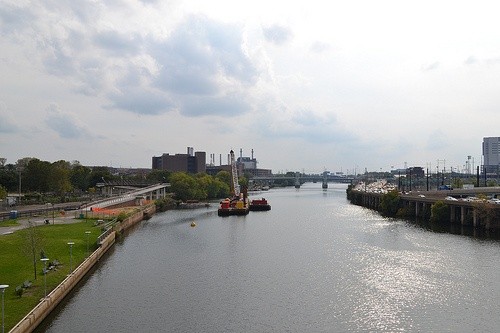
top-left (248, 197), bottom-right (271, 212)
top-left (218, 198), bottom-right (249, 217)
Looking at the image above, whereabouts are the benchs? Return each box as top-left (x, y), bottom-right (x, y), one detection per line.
top-left (15, 281), bottom-right (32, 291)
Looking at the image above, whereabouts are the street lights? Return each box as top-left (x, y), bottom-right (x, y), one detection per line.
top-left (39, 257), bottom-right (51, 296)
top-left (52, 203), bottom-right (54, 224)
top-left (0, 284), bottom-right (10, 333)
top-left (97, 219), bottom-right (104, 234)
top-left (67, 242), bottom-right (75, 271)
top-left (84, 231), bottom-right (92, 256)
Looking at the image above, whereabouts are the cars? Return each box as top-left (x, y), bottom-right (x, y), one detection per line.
top-left (445, 195), bottom-right (500, 204)
